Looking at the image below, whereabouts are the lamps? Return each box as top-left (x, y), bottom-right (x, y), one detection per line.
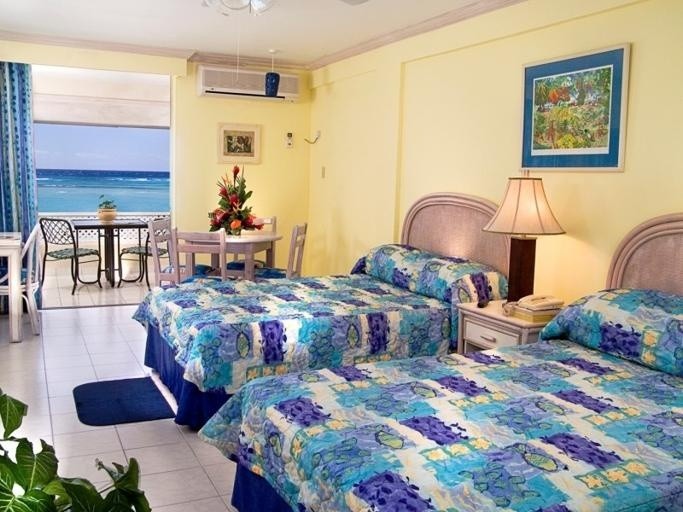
top-left (483, 169), bottom-right (566, 308)
top-left (265, 48), bottom-right (280, 96)
top-left (203, 0), bottom-right (278, 17)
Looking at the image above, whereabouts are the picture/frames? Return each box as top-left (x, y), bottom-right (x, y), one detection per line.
top-left (520, 43), bottom-right (630, 173)
top-left (217, 122), bottom-right (260, 163)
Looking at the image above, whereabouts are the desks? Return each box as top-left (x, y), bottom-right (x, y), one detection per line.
top-left (71, 219), bottom-right (148, 287)
top-left (0, 232), bottom-right (23, 342)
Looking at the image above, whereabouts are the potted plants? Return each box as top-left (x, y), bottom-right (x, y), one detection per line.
top-left (97, 194), bottom-right (116, 223)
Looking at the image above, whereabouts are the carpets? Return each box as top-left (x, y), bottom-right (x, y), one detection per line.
top-left (73, 376), bottom-right (176, 426)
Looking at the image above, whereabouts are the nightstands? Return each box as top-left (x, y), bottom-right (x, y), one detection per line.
top-left (458, 299), bottom-right (549, 354)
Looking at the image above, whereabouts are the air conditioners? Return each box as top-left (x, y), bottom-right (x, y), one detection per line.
top-left (197, 64), bottom-right (299, 104)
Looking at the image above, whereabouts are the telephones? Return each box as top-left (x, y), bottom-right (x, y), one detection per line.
top-left (503, 295), bottom-right (564, 315)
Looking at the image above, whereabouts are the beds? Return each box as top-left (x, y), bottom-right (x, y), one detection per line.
top-left (132, 192), bottom-right (511, 434)
top-left (198, 212), bottom-right (683, 512)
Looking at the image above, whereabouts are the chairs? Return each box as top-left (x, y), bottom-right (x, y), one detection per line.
top-left (40, 217), bottom-right (102, 295)
top-left (117, 218), bottom-right (170, 291)
top-left (148, 215), bottom-right (307, 286)
top-left (0, 223), bottom-right (42, 335)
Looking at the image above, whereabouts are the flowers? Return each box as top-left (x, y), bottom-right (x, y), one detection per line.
top-left (207, 164), bottom-right (265, 233)
